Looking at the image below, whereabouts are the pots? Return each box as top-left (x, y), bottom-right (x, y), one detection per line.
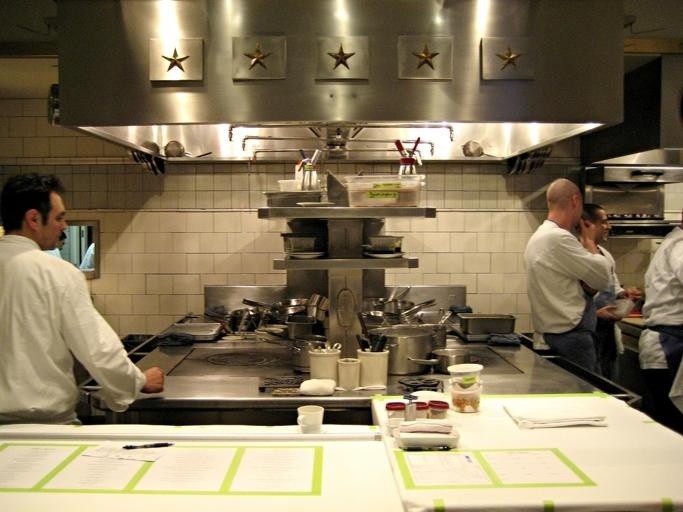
top-left (289, 335), bottom-right (328, 373)
top-left (408, 349), bottom-right (470, 372)
top-left (358, 298), bottom-right (437, 326)
top-left (255, 306), bottom-right (305, 322)
top-left (242, 298), bottom-right (308, 314)
top-left (369, 327), bottom-right (434, 375)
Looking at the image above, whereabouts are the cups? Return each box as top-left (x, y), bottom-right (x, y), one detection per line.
top-left (297, 406), bottom-right (324, 434)
top-left (357, 348), bottom-right (389, 390)
top-left (308, 349), bottom-right (340, 387)
top-left (337, 358), bottom-right (361, 390)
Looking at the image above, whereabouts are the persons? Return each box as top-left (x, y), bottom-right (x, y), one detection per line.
top-left (525, 179), bottom-right (612, 376)
top-left (576, 204), bottom-right (646, 382)
top-left (639, 207), bottom-right (683, 433)
top-left (0, 172), bottom-right (164, 425)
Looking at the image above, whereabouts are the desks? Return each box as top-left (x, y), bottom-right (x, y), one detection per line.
top-left (618, 317), bottom-right (648, 389)
top-left (0, 421), bottom-right (400, 512)
top-left (79, 287), bottom-right (638, 423)
top-left (371, 392), bottom-right (682, 512)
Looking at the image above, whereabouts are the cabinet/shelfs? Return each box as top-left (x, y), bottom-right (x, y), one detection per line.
top-left (258, 206), bottom-right (435, 357)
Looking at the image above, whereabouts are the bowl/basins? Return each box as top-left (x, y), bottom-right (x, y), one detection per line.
top-left (281, 233), bottom-right (320, 253)
top-left (263, 191), bottom-right (322, 207)
top-left (344, 174), bottom-right (427, 189)
top-left (420, 324), bottom-right (447, 349)
top-left (369, 236), bottom-right (403, 252)
top-left (345, 182), bottom-right (426, 207)
top-left (456, 313), bottom-right (519, 334)
top-left (285, 315), bottom-right (316, 339)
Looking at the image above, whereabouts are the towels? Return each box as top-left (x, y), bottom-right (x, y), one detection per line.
top-left (397, 418), bottom-right (460, 448)
top-left (505, 397), bottom-right (611, 428)
top-left (300, 378), bottom-right (337, 395)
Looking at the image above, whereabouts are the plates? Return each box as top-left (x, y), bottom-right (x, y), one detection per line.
top-left (254, 327), bottom-right (283, 335)
top-left (289, 252), bottom-right (322, 259)
top-left (296, 202), bottom-right (335, 206)
top-left (451, 326), bottom-right (518, 342)
top-left (159, 322), bottom-right (222, 341)
top-left (366, 252), bottom-right (406, 258)
